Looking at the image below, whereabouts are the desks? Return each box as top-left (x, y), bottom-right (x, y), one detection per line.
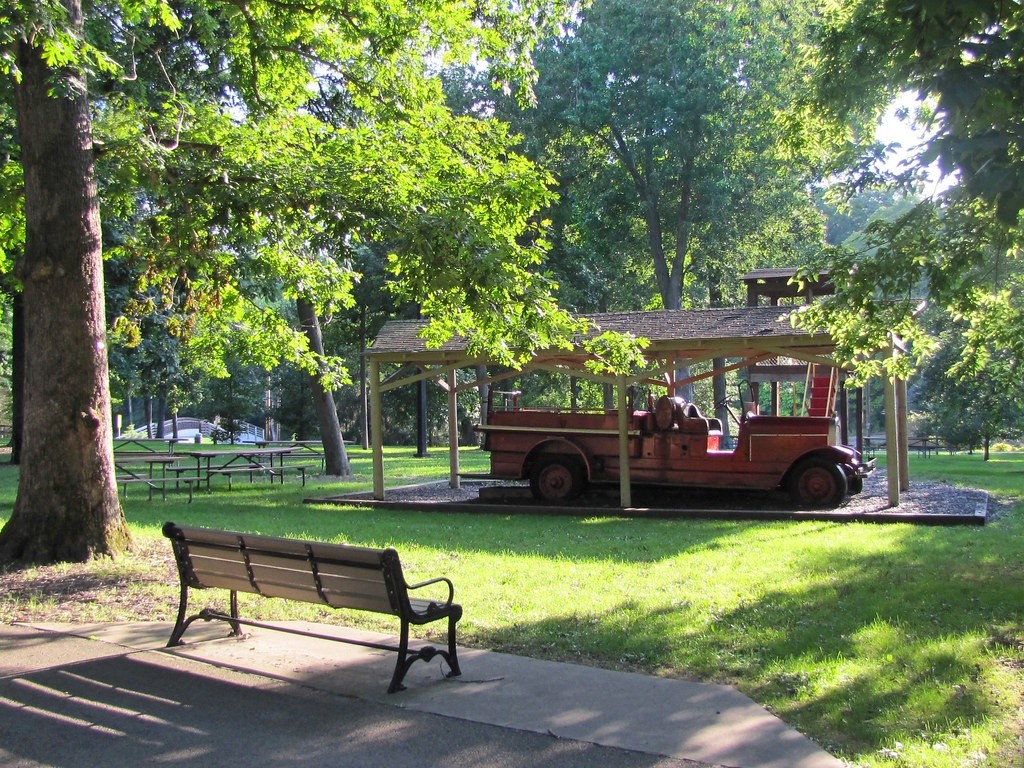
top-left (188, 447), bottom-right (303, 488)
top-left (862, 436), bottom-right (951, 459)
top-left (115, 456), bottom-right (188, 503)
top-left (242, 439), bottom-right (356, 463)
top-left (113, 438), bottom-right (190, 468)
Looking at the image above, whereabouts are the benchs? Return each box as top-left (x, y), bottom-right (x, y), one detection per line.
top-left (862, 443), bottom-right (957, 450)
top-left (677, 403), bottom-right (724, 437)
top-left (161, 521), bottom-right (464, 695)
top-left (114, 448), bottom-right (364, 505)
